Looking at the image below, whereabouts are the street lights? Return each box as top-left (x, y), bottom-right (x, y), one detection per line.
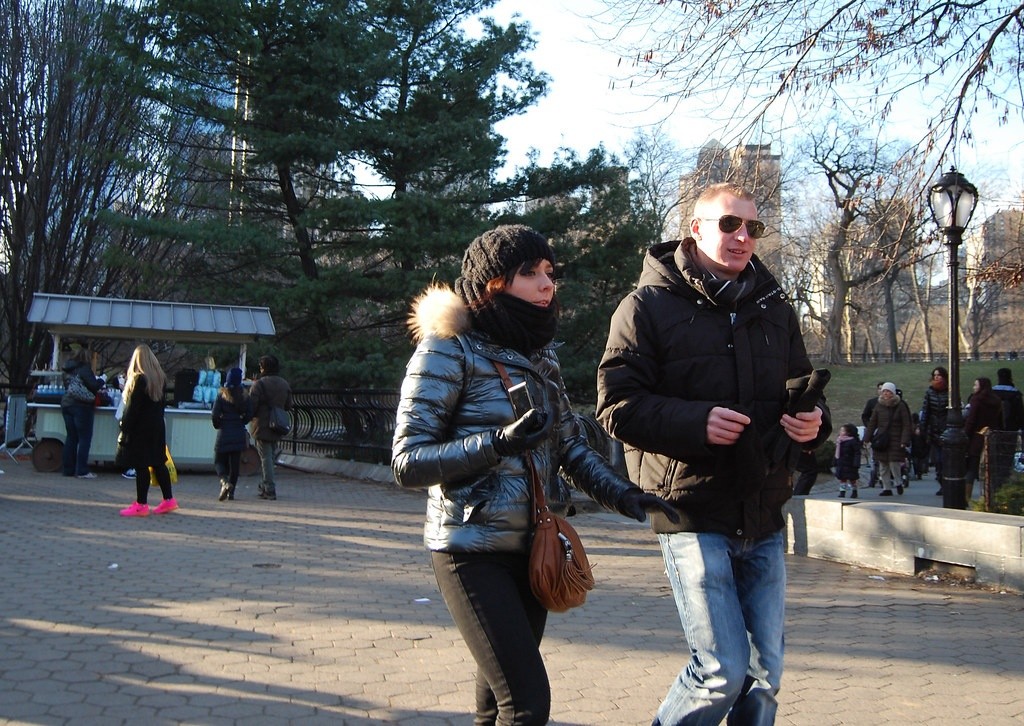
top-left (926, 167), bottom-right (978, 511)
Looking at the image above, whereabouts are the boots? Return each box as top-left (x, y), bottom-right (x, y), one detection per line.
top-left (218, 476), bottom-right (234, 501)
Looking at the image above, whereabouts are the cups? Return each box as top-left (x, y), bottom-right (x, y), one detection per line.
top-left (98, 389), bottom-right (122, 407)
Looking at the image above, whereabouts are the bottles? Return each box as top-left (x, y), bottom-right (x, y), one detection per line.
top-left (37, 385), bottom-right (65, 395)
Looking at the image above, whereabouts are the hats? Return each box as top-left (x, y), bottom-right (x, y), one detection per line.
top-left (226, 367), bottom-right (243, 384)
top-left (946, 408), bottom-right (964, 427)
top-left (881, 382), bottom-right (896, 395)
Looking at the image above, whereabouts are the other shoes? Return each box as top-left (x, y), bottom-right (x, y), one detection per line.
top-left (153, 498), bottom-right (179, 514)
top-left (119, 501), bottom-right (148, 516)
top-left (851, 490), bottom-right (857, 497)
top-left (76, 471), bottom-right (98, 479)
top-left (936, 488), bottom-right (944, 496)
top-left (122, 467), bottom-right (137, 479)
top-left (257, 489), bottom-right (276, 500)
top-left (868, 470), bottom-right (922, 496)
top-left (838, 491), bottom-right (846, 497)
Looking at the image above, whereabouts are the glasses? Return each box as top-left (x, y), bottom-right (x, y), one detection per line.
top-left (701, 214), bottom-right (767, 239)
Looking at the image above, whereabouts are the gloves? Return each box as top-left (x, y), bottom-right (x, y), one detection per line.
top-left (779, 368), bottom-right (831, 441)
top-left (616, 489), bottom-right (681, 524)
top-left (491, 409), bottom-right (554, 456)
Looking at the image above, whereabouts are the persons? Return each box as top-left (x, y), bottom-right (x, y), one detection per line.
top-left (595, 181), bottom-right (833, 726)
top-left (61, 348), bottom-right (108, 479)
top-left (212, 368), bottom-right (251, 501)
top-left (392, 225), bottom-right (681, 726)
top-left (791, 366), bottom-right (1024, 499)
top-left (247, 355), bottom-right (293, 500)
top-left (113, 345), bottom-right (179, 516)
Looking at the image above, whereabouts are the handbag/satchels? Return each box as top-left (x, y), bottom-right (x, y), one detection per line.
top-left (68, 377), bottom-right (96, 403)
top-left (871, 431), bottom-right (890, 452)
top-left (269, 406), bottom-right (290, 436)
top-left (529, 510), bottom-right (596, 615)
top-left (147, 444), bottom-right (178, 487)
